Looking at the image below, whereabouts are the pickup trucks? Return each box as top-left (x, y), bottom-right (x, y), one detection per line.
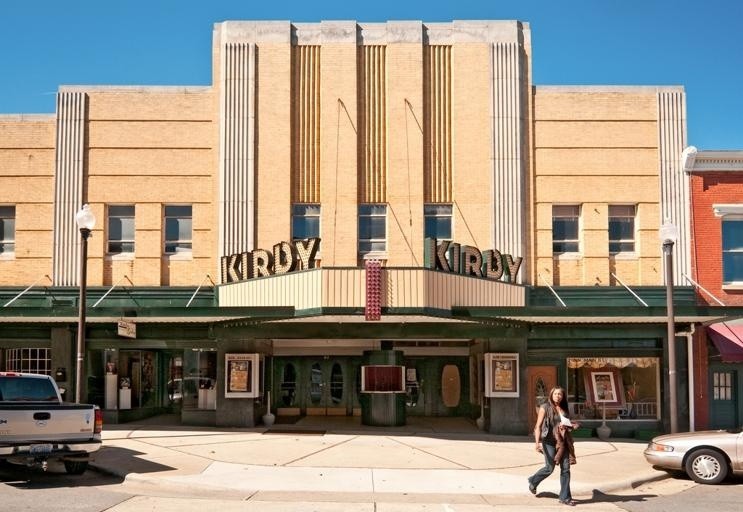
top-left (0, 372), bottom-right (103, 475)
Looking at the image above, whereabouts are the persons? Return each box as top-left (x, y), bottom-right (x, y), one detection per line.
top-left (527, 384), bottom-right (580, 506)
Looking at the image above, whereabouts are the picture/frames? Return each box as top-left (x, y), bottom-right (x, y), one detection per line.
top-left (582, 365), bottom-right (628, 410)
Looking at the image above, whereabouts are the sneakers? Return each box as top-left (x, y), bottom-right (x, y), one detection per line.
top-left (558, 498), bottom-right (576, 506)
top-left (528, 479), bottom-right (537, 494)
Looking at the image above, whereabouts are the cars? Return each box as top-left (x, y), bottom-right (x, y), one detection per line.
top-left (643, 427), bottom-right (743, 484)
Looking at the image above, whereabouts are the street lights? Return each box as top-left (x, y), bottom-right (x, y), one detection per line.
top-left (76, 204), bottom-right (96, 404)
top-left (658, 217), bottom-right (679, 434)
